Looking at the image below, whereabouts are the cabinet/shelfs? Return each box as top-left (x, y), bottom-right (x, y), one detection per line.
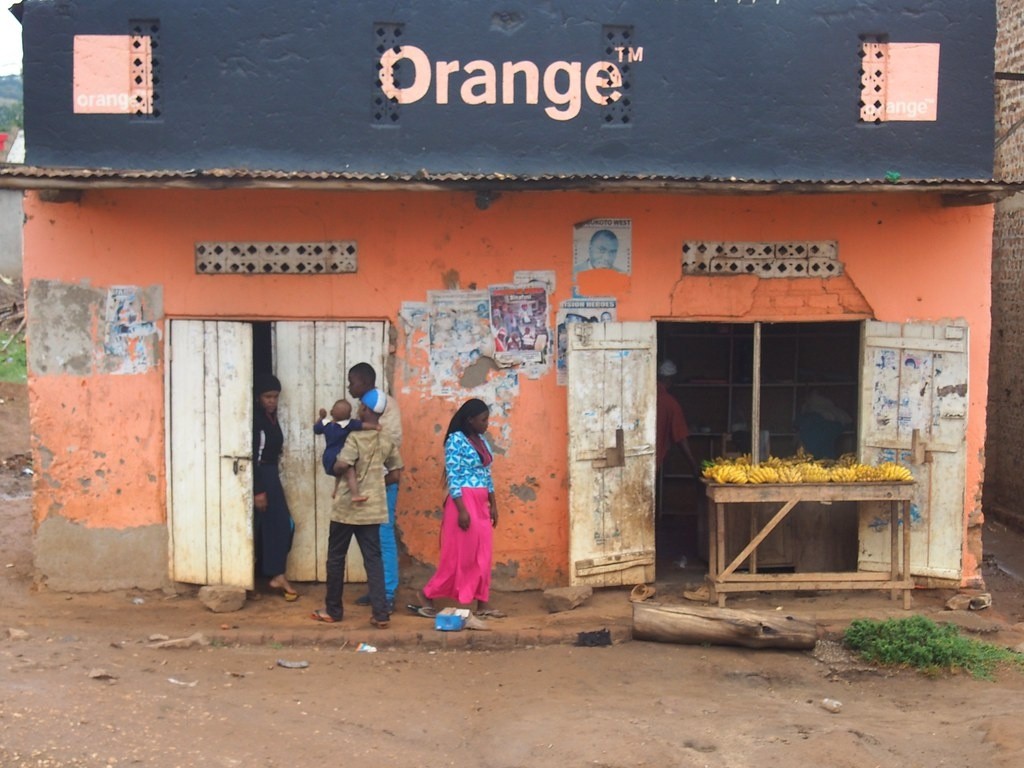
top-left (656, 323), bottom-right (858, 521)
top-left (699, 480), bottom-right (858, 572)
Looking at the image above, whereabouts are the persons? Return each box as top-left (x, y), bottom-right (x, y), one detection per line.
top-left (492, 299), bottom-right (545, 350)
top-left (312, 389), bottom-right (405, 628)
top-left (601, 312), bottom-right (612, 322)
top-left (656, 352), bottom-right (698, 478)
top-left (248, 373), bottom-right (297, 598)
top-left (314, 400), bottom-right (382, 502)
top-left (590, 230), bottom-right (618, 269)
top-left (348, 363), bottom-right (402, 613)
top-left (407, 398), bottom-right (506, 617)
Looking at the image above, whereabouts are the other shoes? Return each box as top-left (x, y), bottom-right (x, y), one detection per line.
top-left (284, 591), bottom-right (297, 601)
top-left (386, 599), bottom-right (394, 615)
top-left (353, 592), bottom-right (371, 606)
top-left (247, 593), bottom-right (263, 601)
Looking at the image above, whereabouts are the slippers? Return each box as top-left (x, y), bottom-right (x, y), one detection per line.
top-left (370, 616), bottom-right (389, 629)
top-left (629, 583), bottom-right (656, 603)
top-left (407, 603), bottom-right (438, 617)
top-left (474, 608), bottom-right (508, 618)
top-left (683, 584), bottom-right (728, 602)
top-left (310, 610), bottom-right (335, 623)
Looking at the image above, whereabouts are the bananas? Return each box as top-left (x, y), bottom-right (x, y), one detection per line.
top-left (701, 446), bottom-right (914, 482)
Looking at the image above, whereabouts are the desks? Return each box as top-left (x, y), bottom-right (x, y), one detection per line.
top-left (698, 477), bottom-right (918, 610)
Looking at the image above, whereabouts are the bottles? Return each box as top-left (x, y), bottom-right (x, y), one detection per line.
top-left (821, 698), bottom-right (842, 713)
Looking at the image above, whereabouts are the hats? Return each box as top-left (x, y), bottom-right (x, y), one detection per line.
top-left (658, 358), bottom-right (677, 375)
top-left (359, 388), bottom-right (387, 414)
top-left (254, 374), bottom-right (281, 398)
